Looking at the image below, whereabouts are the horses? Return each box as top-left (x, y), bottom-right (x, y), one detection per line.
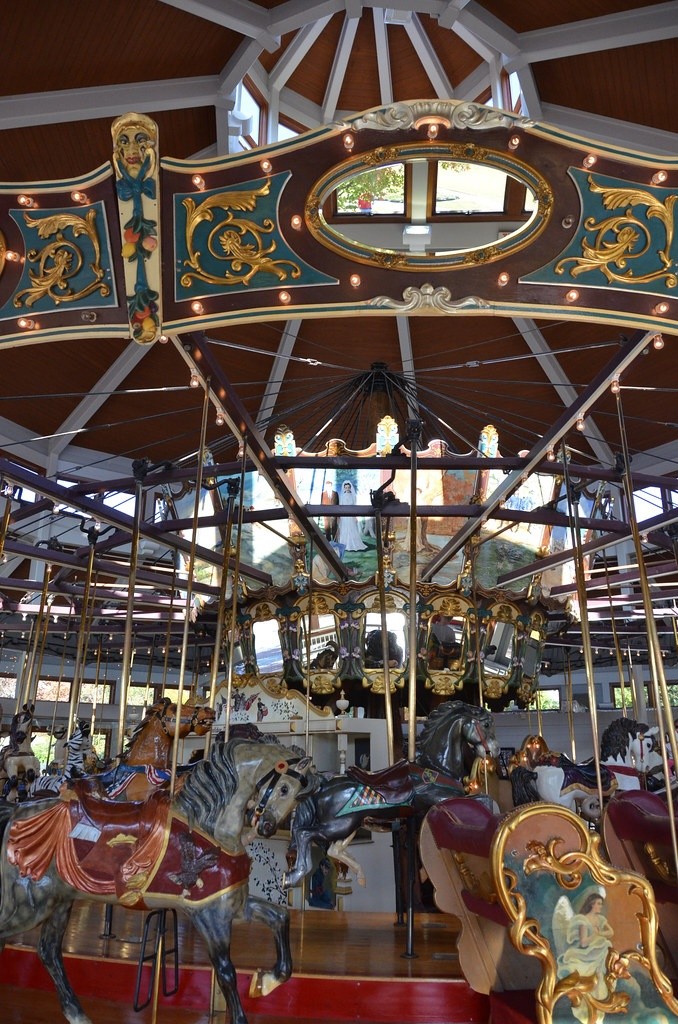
top-left (0, 701), bottom-right (678, 1024)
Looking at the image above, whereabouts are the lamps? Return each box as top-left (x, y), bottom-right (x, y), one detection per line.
top-left (336, 689), bottom-right (350, 714)
